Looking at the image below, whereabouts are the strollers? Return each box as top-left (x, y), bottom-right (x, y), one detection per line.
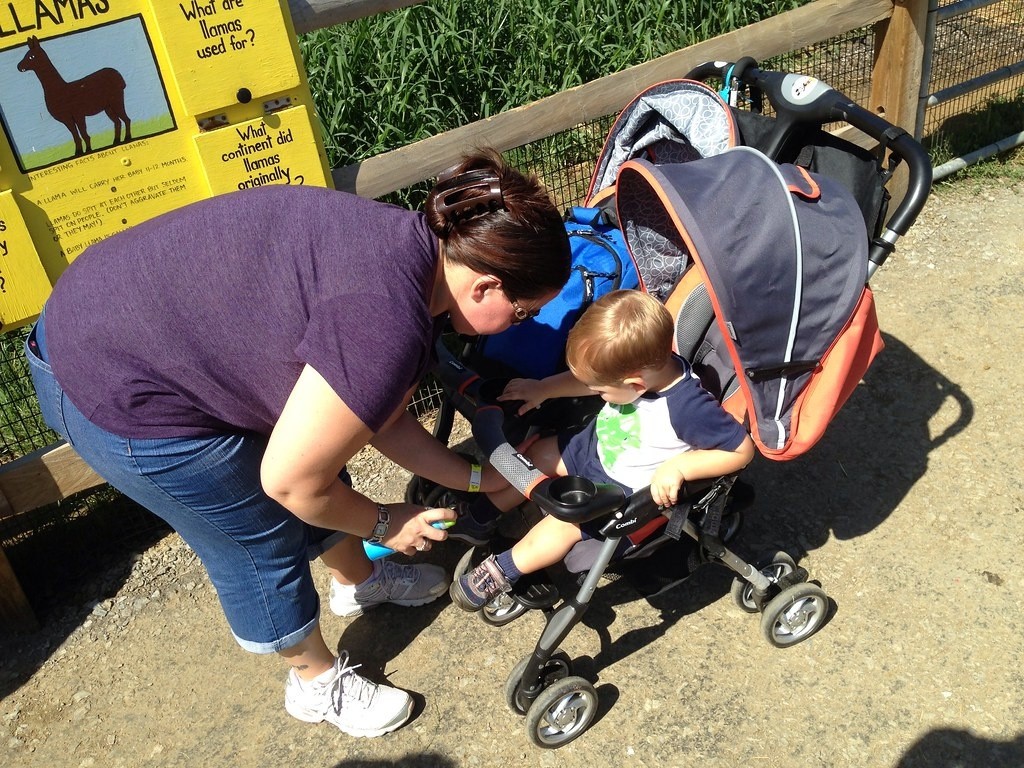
top-left (405, 59), bottom-right (933, 750)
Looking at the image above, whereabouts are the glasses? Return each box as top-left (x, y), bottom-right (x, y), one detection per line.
top-left (507, 294), bottom-right (541, 326)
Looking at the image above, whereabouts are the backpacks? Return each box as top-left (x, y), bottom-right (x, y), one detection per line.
top-left (483, 206), bottom-right (638, 388)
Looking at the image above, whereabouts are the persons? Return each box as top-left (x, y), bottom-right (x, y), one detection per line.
top-left (407, 288), bottom-right (755, 612)
top-left (24, 147), bottom-right (573, 739)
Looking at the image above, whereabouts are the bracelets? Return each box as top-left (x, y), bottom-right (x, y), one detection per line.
top-left (468, 464), bottom-right (482, 492)
top-left (364, 503), bottom-right (391, 544)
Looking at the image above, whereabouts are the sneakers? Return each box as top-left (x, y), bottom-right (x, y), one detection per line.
top-left (329, 558), bottom-right (449, 617)
top-left (284, 650), bottom-right (415, 738)
top-left (449, 553), bottom-right (519, 614)
top-left (444, 501), bottom-right (494, 546)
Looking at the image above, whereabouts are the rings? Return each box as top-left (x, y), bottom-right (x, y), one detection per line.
top-left (416, 541), bottom-right (427, 552)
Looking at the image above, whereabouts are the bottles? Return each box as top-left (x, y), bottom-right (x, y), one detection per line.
top-left (363, 517), bottom-right (455, 561)
